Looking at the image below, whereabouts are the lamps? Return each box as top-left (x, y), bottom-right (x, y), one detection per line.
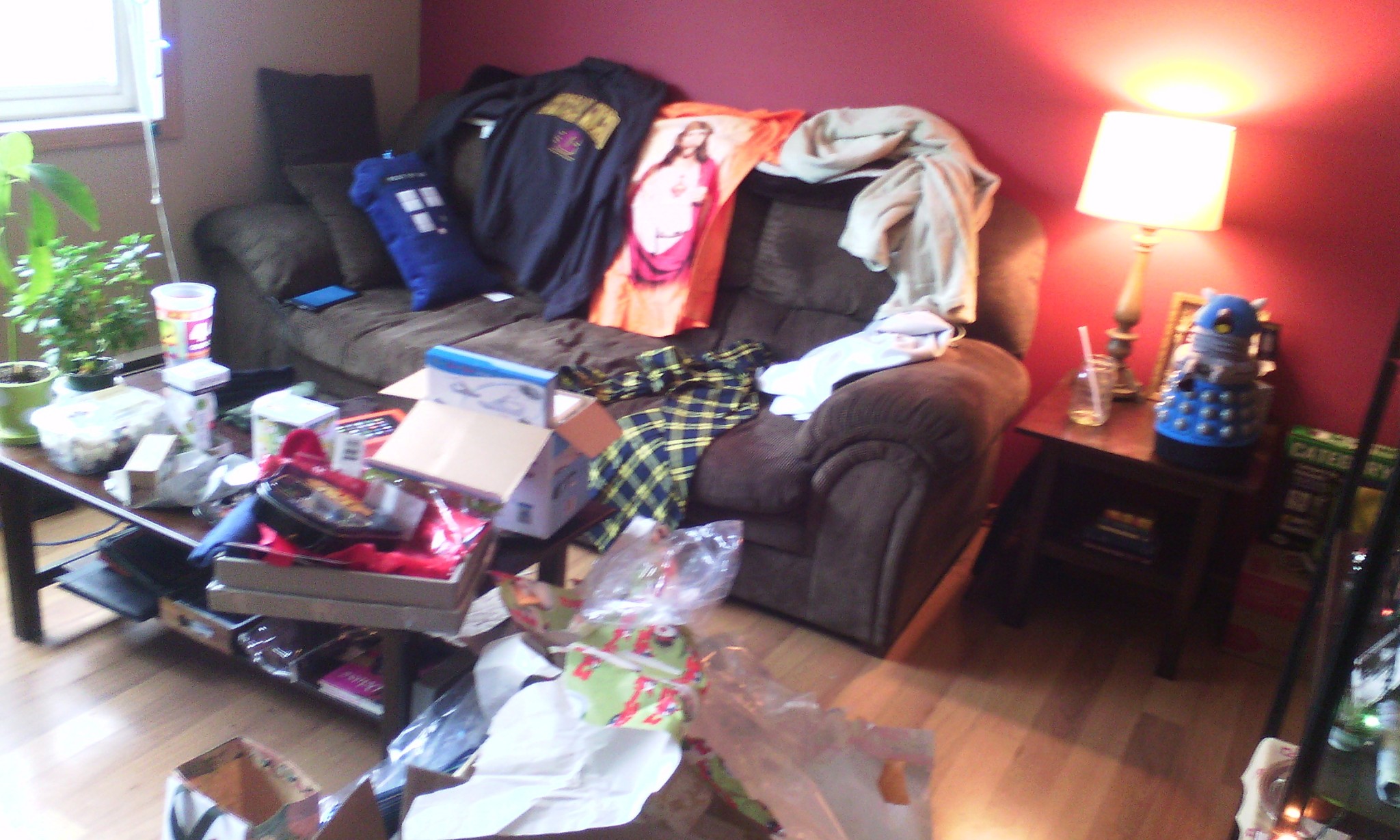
top-left (1074, 108), bottom-right (1239, 400)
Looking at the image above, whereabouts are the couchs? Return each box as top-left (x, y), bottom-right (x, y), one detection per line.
top-left (188, 54), bottom-right (1048, 660)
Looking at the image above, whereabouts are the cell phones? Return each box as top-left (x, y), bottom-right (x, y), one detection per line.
top-left (282, 284), bottom-right (363, 312)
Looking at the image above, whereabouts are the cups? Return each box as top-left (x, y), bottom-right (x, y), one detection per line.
top-left (150, 282), bottom-right (217, 367)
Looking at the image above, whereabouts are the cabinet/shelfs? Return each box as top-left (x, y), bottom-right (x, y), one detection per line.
top-left (1225, 310), bottom-right (1400, 840)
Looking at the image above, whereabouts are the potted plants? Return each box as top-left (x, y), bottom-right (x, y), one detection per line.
top-left (0, 125), bottom-right (164, 446)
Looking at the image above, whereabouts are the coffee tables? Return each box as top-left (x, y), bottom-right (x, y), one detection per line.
top-left (0, 334), bottom-right (624, 764)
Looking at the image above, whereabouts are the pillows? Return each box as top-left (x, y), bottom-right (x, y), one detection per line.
top-left (255, 66), bottom-right (381, 206)
top-left (280, 157), bottom-right (406, 293)
top-left (351, 145), bottom-right (510, 313)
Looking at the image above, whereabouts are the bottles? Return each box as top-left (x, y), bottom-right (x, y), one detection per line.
top-left (1066, 352), bottom-right (1121, 426)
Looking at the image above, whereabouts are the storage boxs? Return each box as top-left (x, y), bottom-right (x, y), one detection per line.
top-left (159, 356), bottom-right (232, 395)
top-left (1267, 423), bottom-right (1400, 560)
top-left (203, 531), bottom-right (500, 636)
top-left (247, 391), bottom-right (341, 466)
top-left (424, 344), bottom-right (585, 429)
top-left (154, 574), bottom-right (270, 658)
top-left (1221, 527), bottom-right (1316, 671)
top-left (362, 362), bottom-right (624, 538)
top-left (212, 500), bottom-right (503, 607)
top-left (126, 431), bottom-right (181, 507)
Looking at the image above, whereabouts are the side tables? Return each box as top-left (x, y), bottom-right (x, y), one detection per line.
top-left (1004, 365), bottom-right (1286, 683)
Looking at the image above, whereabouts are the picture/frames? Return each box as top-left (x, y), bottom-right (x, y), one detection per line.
top-left (1147, 290), bottom-right (1272, 403)
